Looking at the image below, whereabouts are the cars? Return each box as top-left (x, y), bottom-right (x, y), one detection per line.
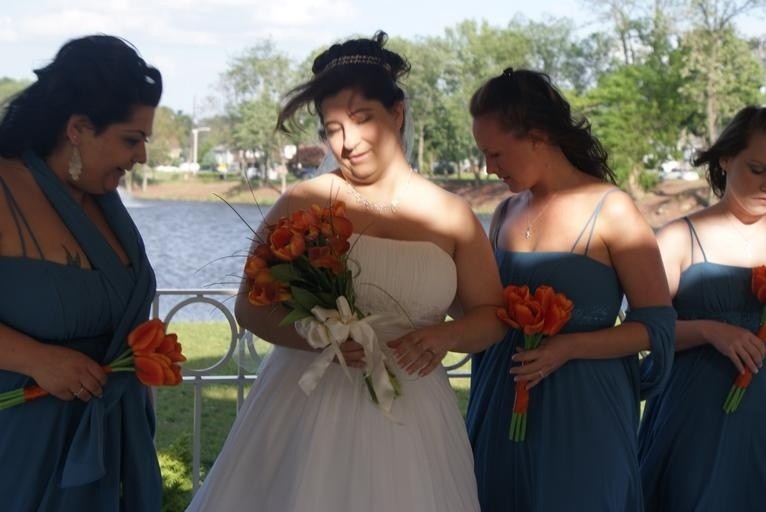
top-left (411, 154), bottom-right (489, 174)
top-left (149, 141), bottom-right (326, 182)
top-left (640, 145), bottom-right (711, 181)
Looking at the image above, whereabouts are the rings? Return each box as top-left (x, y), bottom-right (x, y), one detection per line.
top-left (73, 388), bottom-right (84, 396)
top-left (538, 370), bottom-right (544, 377)
top-left (427, 347), bottom-right (437, 357)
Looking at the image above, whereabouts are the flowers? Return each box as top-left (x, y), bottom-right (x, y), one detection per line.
top-left (721, 264), bottom-right (766, 413)
top-left (495, 283), bottom-right (574, 441)
top-left (0, 318), bottom-right (188, 410)
top-left (200, 177), bottom-right (422, 412)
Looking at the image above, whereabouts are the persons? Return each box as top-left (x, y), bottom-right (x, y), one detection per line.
top-left (654, 105), bottom-right (766, 512)
top-left (1, 33), bottom-right (168, 512)
top-left (180, 30), bottom-right (510, 512)
top-left (465, 64), bottom-right (679, 511)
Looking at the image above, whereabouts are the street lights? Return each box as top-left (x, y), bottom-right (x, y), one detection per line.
top-left (189, 126), bottom-right (211, 166)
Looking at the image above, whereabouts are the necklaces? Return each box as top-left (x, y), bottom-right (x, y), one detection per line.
top-left (524, 172), bottom-right (577, 239)
top-left (343, 169), bottom-right (417, 216)
